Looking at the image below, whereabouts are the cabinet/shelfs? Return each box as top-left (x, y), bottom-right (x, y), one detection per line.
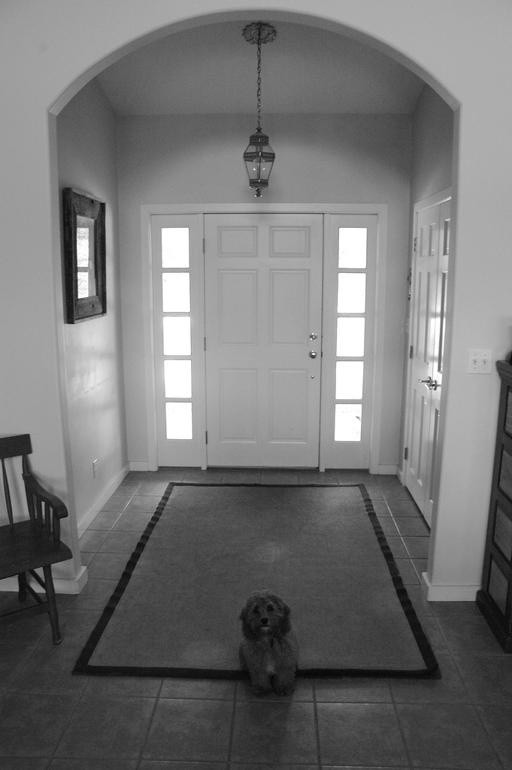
top-left (474, 352), bottom-right (512, 654)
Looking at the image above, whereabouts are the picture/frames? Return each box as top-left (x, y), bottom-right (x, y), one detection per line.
top-left (63, 187), bottom-right (108, 323)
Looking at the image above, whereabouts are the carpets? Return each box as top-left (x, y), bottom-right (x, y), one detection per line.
top-left (70, 481), bottom-right (442, 681)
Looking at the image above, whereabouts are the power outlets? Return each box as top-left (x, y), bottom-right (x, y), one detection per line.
top-left (466, 348), bottom-right (493, 374)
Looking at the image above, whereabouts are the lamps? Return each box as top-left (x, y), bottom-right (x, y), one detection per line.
top-left (241, 21), bottom-right (277, 199)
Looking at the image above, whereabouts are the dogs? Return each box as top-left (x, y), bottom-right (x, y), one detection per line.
top-left (236, 590), bottom-right (299, 698)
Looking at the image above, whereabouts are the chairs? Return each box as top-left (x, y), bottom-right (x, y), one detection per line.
top-left (0, 434), bottom-right (73, 646)
